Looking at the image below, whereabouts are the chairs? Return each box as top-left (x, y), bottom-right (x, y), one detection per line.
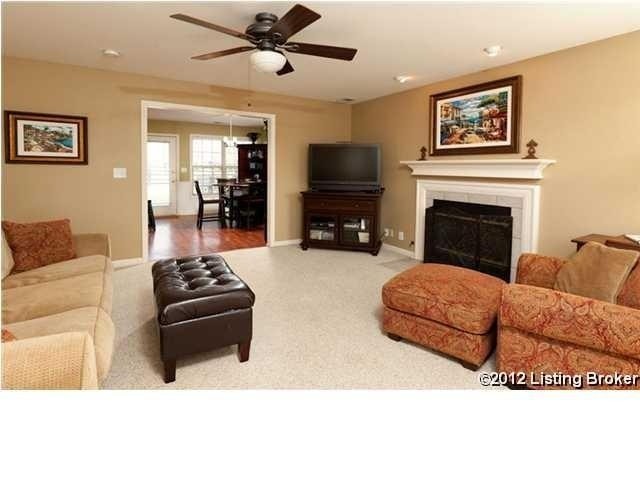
top-left (192, 179), bottom-right (227, 230)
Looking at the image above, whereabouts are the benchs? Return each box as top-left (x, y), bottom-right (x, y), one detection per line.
top-left (149, 254), bottom-right (258, 385)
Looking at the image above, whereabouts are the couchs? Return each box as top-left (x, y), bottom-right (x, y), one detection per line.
top-left (0, 218), bottom-right (115, 389)
top-left (496, 252), bottom-right (640, 390)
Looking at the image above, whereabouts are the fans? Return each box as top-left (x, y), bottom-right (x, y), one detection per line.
top-left (170, 3), bottom-right (358, 79)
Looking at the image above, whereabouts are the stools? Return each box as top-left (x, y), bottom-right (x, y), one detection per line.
top-left (380, 261), bottom-right (505, 371)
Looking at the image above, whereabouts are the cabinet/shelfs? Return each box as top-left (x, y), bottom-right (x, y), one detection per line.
top-left (238, 144), bottom-right (265, 182)
top-left (306, 192), bottom-right (376, 250)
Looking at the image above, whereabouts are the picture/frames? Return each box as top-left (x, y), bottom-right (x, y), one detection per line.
top-left (2, 110), bottom-right (89, 167)
top-left (427, 73), bottom-right (524, 154)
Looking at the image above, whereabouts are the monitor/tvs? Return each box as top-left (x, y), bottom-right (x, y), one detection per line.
top-left (307, 142), bottom-right (381, 192)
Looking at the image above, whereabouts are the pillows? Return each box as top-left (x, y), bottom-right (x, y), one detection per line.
top-left (550, 239), bottom-right (639, 305)
top-left (2, 219), bottom-right (77, 267)
top-left (0, 231), bottom-right (15, 280)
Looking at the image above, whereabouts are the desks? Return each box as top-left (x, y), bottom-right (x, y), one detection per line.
top-left (212, 182), bottom-right (257, 228)
top-left (571, 233), bottom-right (640, 252)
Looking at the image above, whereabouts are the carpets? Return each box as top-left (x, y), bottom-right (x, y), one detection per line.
top-left (100, 241), bottom-right (515, 389)
